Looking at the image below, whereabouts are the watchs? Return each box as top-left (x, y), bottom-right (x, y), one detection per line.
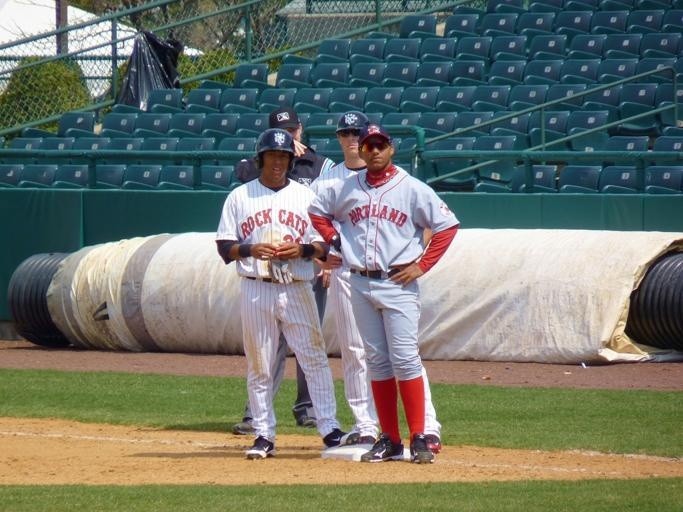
top-left (331, 233), bottom-right (339, 244)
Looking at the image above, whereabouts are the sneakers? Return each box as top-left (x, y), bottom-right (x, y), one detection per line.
top-left (323, 429), bottom-right (360, 447)
top-left (298, 417), bottom-right (317, 428)
top-left (410, 433), bottom-right (434, 464)
top-left (231, 418), bottom-right (258, 434)
top-left (425, 434), bottom-right (440, 454)
top-left (246, 437), bottom-right (276, 459)
top-left (361, 433), bottom-right (404, 462)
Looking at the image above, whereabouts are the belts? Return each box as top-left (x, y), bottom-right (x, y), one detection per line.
top-left (247, 276), bottom-right (271, 282)
top-left (350, 267), bottom-right (398, 279)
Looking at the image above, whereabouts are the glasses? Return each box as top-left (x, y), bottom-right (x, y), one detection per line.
top-left (366, 142), bottom-right (385, 152)
top-left (340, 130), bottom-right (358, 137)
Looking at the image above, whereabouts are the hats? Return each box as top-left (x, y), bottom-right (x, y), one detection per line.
top-left (358, 124), bottom-right (391, 146)
top-left (269, 109), bottom-right (297, 129)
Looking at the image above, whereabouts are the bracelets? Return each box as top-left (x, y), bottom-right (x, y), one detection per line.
top-left (238, 243), bottom-right (252, 258)
top-left (301, 243), bottom-right (314, 258)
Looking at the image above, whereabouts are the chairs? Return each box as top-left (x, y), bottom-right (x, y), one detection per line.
top-left (0, 1), bottom-right (683, 198)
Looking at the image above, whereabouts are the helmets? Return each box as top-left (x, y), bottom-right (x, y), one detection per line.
top-left (257, 128), bottom-right (293, 170)
top-left (336, 110), bottom-right (370, 131)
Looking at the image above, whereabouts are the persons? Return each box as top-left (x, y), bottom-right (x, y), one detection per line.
top-left (306, 123), bottom-right (460, 466)
top-left (307, 111), bottom-right (443, 453)
top-left (229, 106), bottom-right (337, 436)
top-left (215, 129), bottom-right (361, 461)
